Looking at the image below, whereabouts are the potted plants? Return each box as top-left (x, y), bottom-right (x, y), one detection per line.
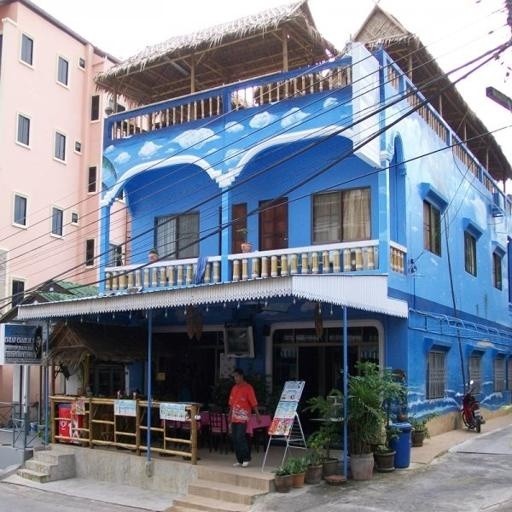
top-left (270, 359), bottom-right (441, 493)
top-left (148, 248), bottom-right (160, 262)
top-left (236, 228), bottom-right (253, 252)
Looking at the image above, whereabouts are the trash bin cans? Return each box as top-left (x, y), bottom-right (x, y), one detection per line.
top-left (384, 422), bottom-right (415, 468)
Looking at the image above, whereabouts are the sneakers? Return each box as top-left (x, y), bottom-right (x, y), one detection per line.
top-left (233, 461), bottom-right (249, 467)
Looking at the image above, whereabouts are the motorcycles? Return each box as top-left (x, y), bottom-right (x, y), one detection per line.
top-left (455, 379), bottom-right (486, 433)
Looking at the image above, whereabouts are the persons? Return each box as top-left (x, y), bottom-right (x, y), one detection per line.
top-left (226, 368), bottom-right (262, 466)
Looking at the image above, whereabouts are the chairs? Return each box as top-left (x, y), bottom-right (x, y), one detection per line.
top-left (175, 398), bottom-right (267, 453)
